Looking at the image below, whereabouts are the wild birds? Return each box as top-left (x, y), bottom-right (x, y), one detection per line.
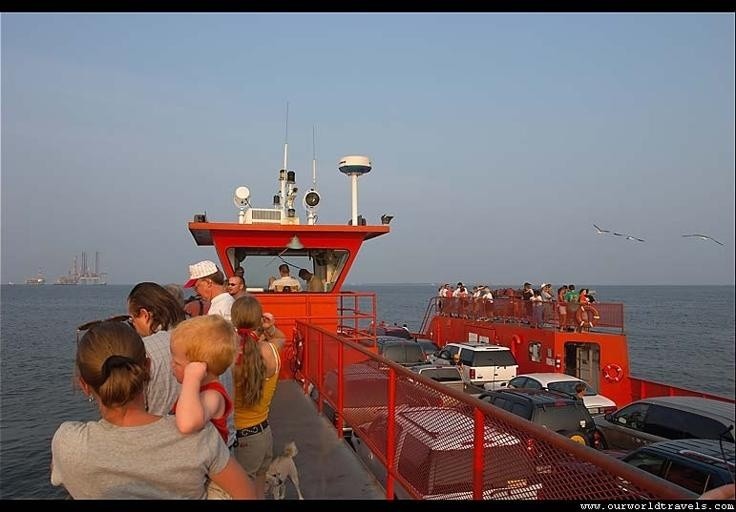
top-left (593, 224), bottom-right (611, 235)
top-left (681, 232), bottom-right (725, 248)
top-left (613, 231), bottom-right (647, 244)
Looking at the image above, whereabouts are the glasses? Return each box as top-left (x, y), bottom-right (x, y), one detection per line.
top-left (76, 315), bottom-right (137, 347)
top-left (228, 283), bottom-right (237, 286)
top-left (192, 283), bottom-right (200, 296)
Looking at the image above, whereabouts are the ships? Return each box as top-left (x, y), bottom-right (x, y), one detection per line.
top-left (56, 249), bottom-right (108, 285)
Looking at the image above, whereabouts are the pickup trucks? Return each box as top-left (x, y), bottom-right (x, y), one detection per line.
top-left (411, 365), bottom-right (490, 402)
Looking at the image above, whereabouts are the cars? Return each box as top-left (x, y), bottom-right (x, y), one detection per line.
top-left (502, 373), bottom-right (618, 414)
top-left (411, 339), bottom-right (443, 358)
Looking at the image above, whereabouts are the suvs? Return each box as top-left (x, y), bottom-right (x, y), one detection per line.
top-left (429, 342), bottom-right (524, 388)
top-left (592, 397), bottom-right (736, 450)
top-left (352, 406), bottom-right (543, 500)
top-left (375, 336), bottom-right (427, 366)
top-left (478, 387), bottom-right (602, 449)
top-left (311, 363), bottom-right (410, 437)
top-left (537, 439), bottom-right (735, 501)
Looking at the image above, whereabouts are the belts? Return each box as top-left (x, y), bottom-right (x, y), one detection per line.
top-left (237, 420), bottom-right (268, 439)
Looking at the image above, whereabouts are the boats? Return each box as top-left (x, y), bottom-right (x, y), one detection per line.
top-left (25, 278), bottom-right (45, 286)
top-left (185, 103), bottom-right (736, 500)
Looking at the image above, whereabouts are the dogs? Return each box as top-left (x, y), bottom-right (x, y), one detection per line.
top-left (263, 440), bottom-right (309, 501)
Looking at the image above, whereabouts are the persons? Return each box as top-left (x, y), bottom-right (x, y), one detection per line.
top-left (380, 321), bottom-right (386, 327)
top-left (297, 268), bottom-right (325, 293)
top-left (437, 281), bottom-right (596, 334)
top-left (266, 264), bottom-right (305, 292)
top-left (126, 257), bottom-right (290, 500)
top-left (449, 354), bottom-right (464, 372)
top-left (574, 383), bottom-right (591, 415)
top-left (46, 311), bottom-right (262, 500)
top-left (393, 323), bottom-right (398, 327)
top-left (401, 323), bottom-right (411, 333)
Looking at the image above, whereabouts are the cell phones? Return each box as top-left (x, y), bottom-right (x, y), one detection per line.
top-left (261, 314), bottom-right (271, 322)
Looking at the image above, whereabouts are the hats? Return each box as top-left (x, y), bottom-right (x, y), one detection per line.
top-left (524, 282), bottom-right (531, 286)
top-left (579, 288), bottom-right (589, 294)
top-left (184, 260), bottom-right (219, 288)
top-left (540, 283), bottom-right (549, 288)
